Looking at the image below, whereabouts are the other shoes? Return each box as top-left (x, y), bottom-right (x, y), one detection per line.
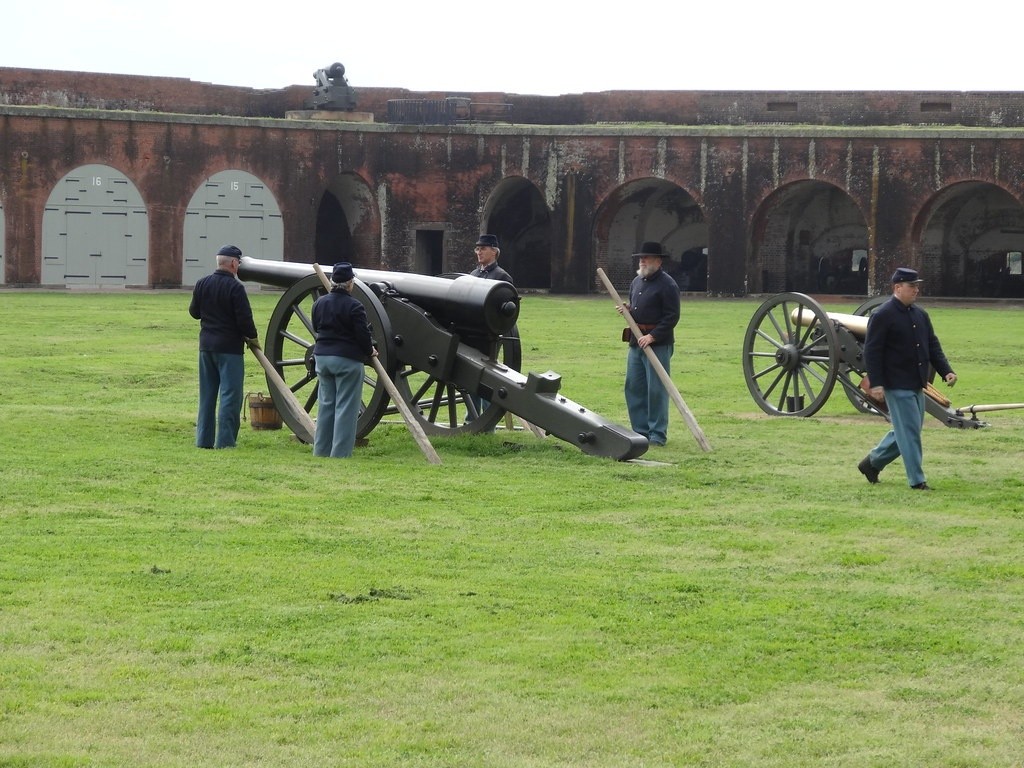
top-left (649, 439), bottom-right (663, 447)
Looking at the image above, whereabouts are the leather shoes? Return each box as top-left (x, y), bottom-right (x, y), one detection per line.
top-left (857, 455), bottom-right (880, 483)
top-left (911, 481), bottom-right (934, 491)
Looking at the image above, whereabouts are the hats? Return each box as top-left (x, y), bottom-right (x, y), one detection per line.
top-left (331, 262), bottom-right (353, 283)
top-left (891, 267), bottom-right (924, 284)
top-left (476, 234), bottom-right (498, 247)
top-left (216, 245), bottom-right (242, 259)
top-left (631, 242), bottom-right (669, 257)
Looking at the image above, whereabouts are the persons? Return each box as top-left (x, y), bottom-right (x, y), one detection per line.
top-left (460, 234), bottom-right (513, 434)
top-left (616, 242), bottom-right (680, 446)
top-left (311, 262), bottom-right (378, 458)
top-left (188, 245), bottom-right (262, 449)
top-left (858, 267), bottom-right (958, 490)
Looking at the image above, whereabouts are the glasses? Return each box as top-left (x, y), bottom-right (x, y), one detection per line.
top-left (235, 259), bottom-right (243, 265)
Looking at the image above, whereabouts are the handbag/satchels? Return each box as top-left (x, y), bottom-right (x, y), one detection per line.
top-left (622, 326), bottom-right (631, 342)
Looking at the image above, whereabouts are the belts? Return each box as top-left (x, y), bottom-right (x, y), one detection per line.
top-left (637, 324), bottom-right (655, 330)
